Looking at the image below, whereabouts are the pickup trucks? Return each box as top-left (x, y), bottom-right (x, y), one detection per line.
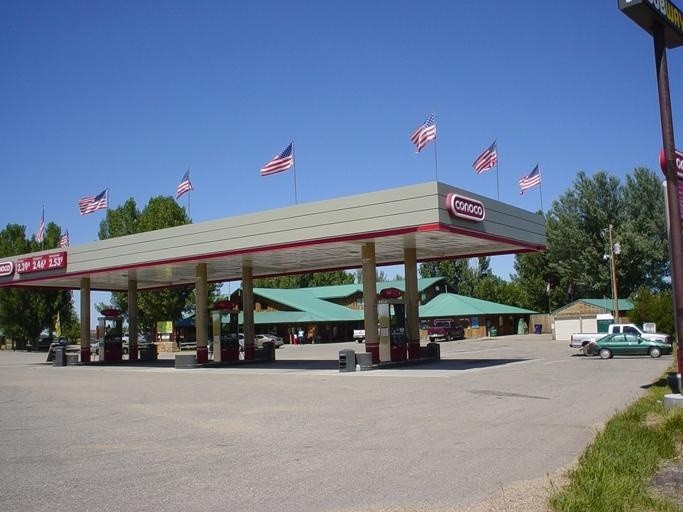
top-left (571, 324), bottom-right (671, 356)
top-left (352, 323), bottom-right (380, 343)
top-left (427, 320), bottom-right (465, 342)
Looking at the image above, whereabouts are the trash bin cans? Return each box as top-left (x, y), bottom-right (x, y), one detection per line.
top-left (535, 324), bottom-right (542, 334)
top-left (55, 346), bottom-right (66, 367)
top-left (339, 349), bottom-right (355, 372)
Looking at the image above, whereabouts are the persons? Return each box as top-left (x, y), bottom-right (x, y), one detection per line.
top-left (95, 325), bottom-right (99, 339)
top-left (106, 324), bottom-right (112, 334)
top-left (297, 327), bottom-right (304, 344)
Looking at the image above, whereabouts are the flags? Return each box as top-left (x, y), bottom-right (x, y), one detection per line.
top-left (57, 233), bottom-right (70, 247)
top-left (518, 162), bottom-right (541, 195)
top-left (470, 139), bottom-right (498, 175)
top-left (545, 281), bottom-right (551, 295)
top-left (175, 170), bottom-right (193, 200)
top-left (260, 141), bottom-right (295, 177)
top-left (568, 284), bottom-right (573, 294)
top-left (409, 113), bottom-right (436, 153)
top-left (78, 189), bottom-right (108, 215)
top-left (35, 214), bottom-right (45, 244)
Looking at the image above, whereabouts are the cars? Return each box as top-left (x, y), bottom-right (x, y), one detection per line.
top-left (91, 330), bottom-right (146, 355)
top-left (210, 333), bottom-right (258, 353)
top-left (254, 334), bottom-right (284, 349)
top-left (589, 333), bottom-right (672, 358)
